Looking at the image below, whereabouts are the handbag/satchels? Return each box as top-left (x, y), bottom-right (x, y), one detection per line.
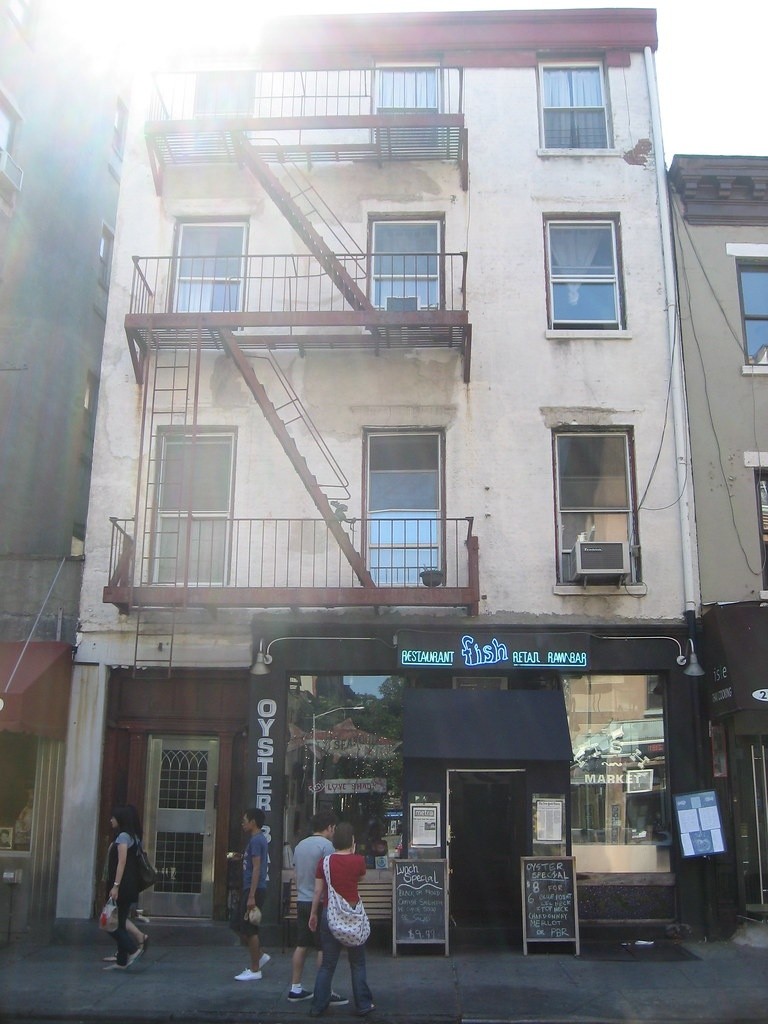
top-left (326, 886), bottom-right (371, 947)
top-left (135, 841), bottom-right (157, 894)
top-left (100, 896), bottom-right (120, 933)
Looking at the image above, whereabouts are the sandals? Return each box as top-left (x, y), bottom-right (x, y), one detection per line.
top-left (138, 933), bottom-right (148, 951)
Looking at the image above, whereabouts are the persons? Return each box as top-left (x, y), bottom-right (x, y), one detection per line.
top-left (234, 807), bottom-right (271, 980)
top-left (286, 814), bottom-right (352, 1007)
top-left (97, 804), bottom-right (159, 972)
top-left (302, 823), bottom-right (378, 1017)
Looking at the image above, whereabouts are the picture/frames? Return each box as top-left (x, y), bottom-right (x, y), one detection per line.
top-left (0, 828), bottom-right (13, 849)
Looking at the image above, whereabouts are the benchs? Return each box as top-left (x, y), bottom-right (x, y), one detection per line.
top-left (279, 878), bottom-right (393, 954)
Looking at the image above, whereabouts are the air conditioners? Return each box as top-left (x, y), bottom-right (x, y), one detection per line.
top-left (569, 541), bottom-right (630, 581)
top-left (384, 295), bottom-right (420, 312)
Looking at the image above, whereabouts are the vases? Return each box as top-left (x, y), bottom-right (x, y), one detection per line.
top-left (420, 570), bottom-right (442, 586)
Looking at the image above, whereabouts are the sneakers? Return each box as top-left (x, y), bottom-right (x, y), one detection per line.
top-left (329, 991), bottom-right (349, 1006)
top-left (288, 989), bottom-right (314, 1001)
top-left (233, 953), bottom-right (271, 982)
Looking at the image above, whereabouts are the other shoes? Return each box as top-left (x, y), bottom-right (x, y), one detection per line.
top-left (103, 949), bottom-right (144, 971)
top-left (355, 1002), bottom-right (378, 1015)
top-left (309, 1005), bottom-right (326, 1016)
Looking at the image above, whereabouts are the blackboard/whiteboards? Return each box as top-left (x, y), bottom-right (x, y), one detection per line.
top-left (520, 856), bottom-right (579, 943)
top-left (392, 858), bottom-right (451, 944)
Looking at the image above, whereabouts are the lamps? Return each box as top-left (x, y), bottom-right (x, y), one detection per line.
top-left (677, 639), bottom-right (705, 676)
top-left (251, 638), bottom-right (273, 675)
